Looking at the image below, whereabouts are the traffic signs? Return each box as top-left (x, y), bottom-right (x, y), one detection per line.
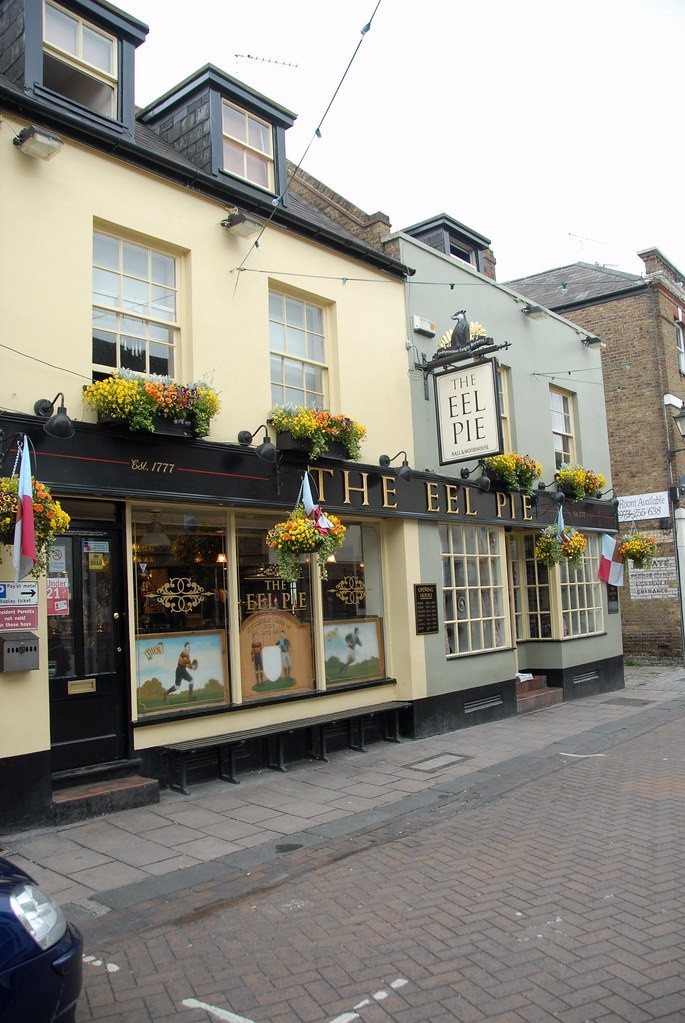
top-left (0, 580), bottom-right (40, 606)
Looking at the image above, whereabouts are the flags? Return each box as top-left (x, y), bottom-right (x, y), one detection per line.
top-left (597, 529), bottom-right (624, 586)
top-left (12, 433), bottom-right (36, 586)
top-left (557, 504), bottom-right (571, 549)
top-left (302, 470), bottom-right (334, 535)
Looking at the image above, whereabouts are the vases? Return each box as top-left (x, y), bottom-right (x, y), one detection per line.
top-left (276, 430), bottom-right (347, 463)
top-left (97, 404), bottom-right (200, 440)
top-left (486, 467), bottom-right (508, 489)
top-left (0, 511), bottom-right (17, 546)
top-left (560, 482), bottom-right (577, 498)
top-left (513, 469), bottom-right (528, 487)
top-left (286, 540), bottom-right (324, 553)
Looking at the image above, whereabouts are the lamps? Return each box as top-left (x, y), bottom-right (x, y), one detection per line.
top-left (34, 392), bottom-right (75, 440)
top-left (538, 480), bottom-right (565, 505)
top-left (216, 553), bottom-right (227, 562)
top-left (326, 554), bottom-right (336, 563)
top-left (460, 463), bottom-right (490, 492)
top-left (138, 513), bottom-right (172, 547)
top-left (221, 213), bottom-right (265, 239)
top-left (237, 425), bottom-right (279, 462)
top-left (521, 304), bottom-right (550, 321)
top-left (13, 125), bottom-right (64, 162)
top-left (379, 451), bottom-right (412, 482)
top-left (595, 488), bottom-right (620, 510)
top-left (581, 336), bottom-right (607, 351)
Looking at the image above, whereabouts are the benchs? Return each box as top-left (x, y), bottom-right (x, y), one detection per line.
top-left (162, 700), bottom-right (414, 797)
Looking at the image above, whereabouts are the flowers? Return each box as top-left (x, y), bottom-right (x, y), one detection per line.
top-left (534, 523), bottom-right (587, 575)
top-left (264, 504), bottom-right (346, 583)
top-left (81, 366), bottom-right (224, 437)
top-left (617, 531), bottom-right (657, 571)
top-left (484, 451), bottom-right (543, 497)
top-left (270, 399), bottom-right (369, 462)
top-left (555, 461), bottom-right (607, 503)
top-left (170, 520), bottom-right (217, 567)
top-left (0, 474), bottom-right (71, 580)
